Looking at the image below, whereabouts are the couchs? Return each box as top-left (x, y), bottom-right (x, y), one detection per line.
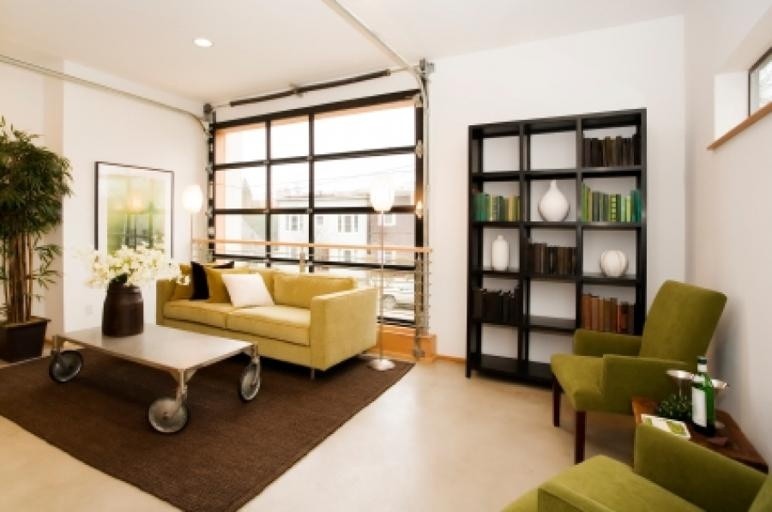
top-left (502, 422), bottom-right (772, 512)
top-left (550, 279), bottom-right (727, 463)
top-left (156, 261), bottom-right (378, 380)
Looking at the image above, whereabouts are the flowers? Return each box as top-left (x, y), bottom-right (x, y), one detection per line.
top-left (85, 240), bottom-right (159, 288)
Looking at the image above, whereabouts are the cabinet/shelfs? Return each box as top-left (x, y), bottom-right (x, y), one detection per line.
top-left (466, 108), bottom-right (647, 388)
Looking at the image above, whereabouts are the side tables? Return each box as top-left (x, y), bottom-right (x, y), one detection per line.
top-left (627, 395), bottom-right (768, 472)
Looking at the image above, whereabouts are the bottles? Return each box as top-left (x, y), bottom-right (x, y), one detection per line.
top-left (691, 358), bottom-right (714, 434)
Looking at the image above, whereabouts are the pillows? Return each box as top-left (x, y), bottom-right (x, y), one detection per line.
top-left (170, 262), bottom-right (275, 308)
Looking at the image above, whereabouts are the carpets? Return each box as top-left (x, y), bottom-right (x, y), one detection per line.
top-left (0, 348), bottom-right (416, 512)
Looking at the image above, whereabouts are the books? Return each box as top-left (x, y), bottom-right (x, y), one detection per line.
top-left (472, 287), bottom-right (520, 320)
top-left (581, 294), bottom-right (635, 335)
top-left (583, 133), bottom-right (640, 167)
top-left (528, 243), bottom-right (577, 275)
top-left (473, 192), bottom-right (520, 222)
top-left (580, 182), bottom-right (641, 222)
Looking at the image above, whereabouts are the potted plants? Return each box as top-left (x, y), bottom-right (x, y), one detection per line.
top-left (0, 114), bottom-right (76, 363)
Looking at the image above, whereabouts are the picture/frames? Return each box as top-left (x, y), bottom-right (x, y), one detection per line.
top-left (93, 161), bottom-right (174, 260)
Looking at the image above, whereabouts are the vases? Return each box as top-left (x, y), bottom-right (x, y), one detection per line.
top-left (537, 180), bottom-right (570, 222)
top-left (103, 287), bottom-right (144, 336)
top-left (600, 248), bottom-right (628, 279)
top-left (492, 235), bottom-right (509, 271)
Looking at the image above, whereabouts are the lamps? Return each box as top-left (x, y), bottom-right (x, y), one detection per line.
top-left (369, 181), bottom-right (396, 371)
top-left (182, 184), bottom-right (203, 261)
top-left (192, 19), bottom-right (214, 48)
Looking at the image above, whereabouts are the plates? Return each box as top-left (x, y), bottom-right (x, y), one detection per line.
top-left (381, 294), bottom-right (397, 310)
